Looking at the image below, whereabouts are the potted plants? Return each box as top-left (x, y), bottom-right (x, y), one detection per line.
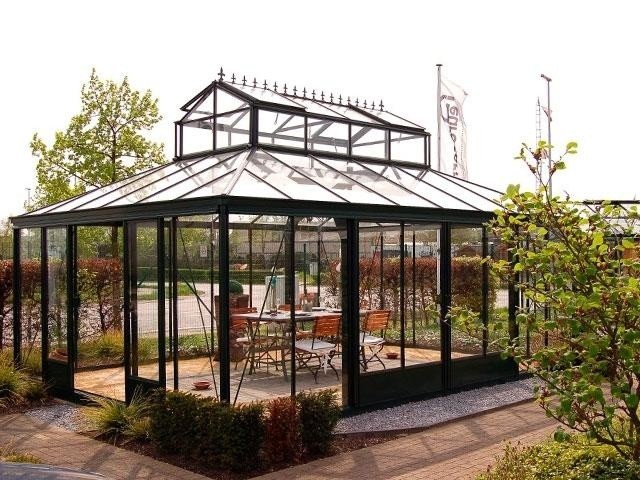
top-left (229, 279), bottom-right (243, 298)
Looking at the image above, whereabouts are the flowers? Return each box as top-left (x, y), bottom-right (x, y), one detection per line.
top-left (300, 292), bottom-right (314, 303)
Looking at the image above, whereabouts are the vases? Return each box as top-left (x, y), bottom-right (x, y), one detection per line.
top-left (303, 304), bottom-right (312, 312)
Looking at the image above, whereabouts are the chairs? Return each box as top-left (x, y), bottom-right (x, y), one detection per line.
top-left (230, 304), bottom-right (391, 383)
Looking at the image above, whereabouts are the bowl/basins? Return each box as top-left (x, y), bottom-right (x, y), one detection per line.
top-left (193, 381), bottom-right (213, 390)
top-left (386, 352), bottom-right (400, 359)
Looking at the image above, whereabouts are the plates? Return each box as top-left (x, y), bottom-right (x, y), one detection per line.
top-left (263, 306), bottom-right (343, 317)
